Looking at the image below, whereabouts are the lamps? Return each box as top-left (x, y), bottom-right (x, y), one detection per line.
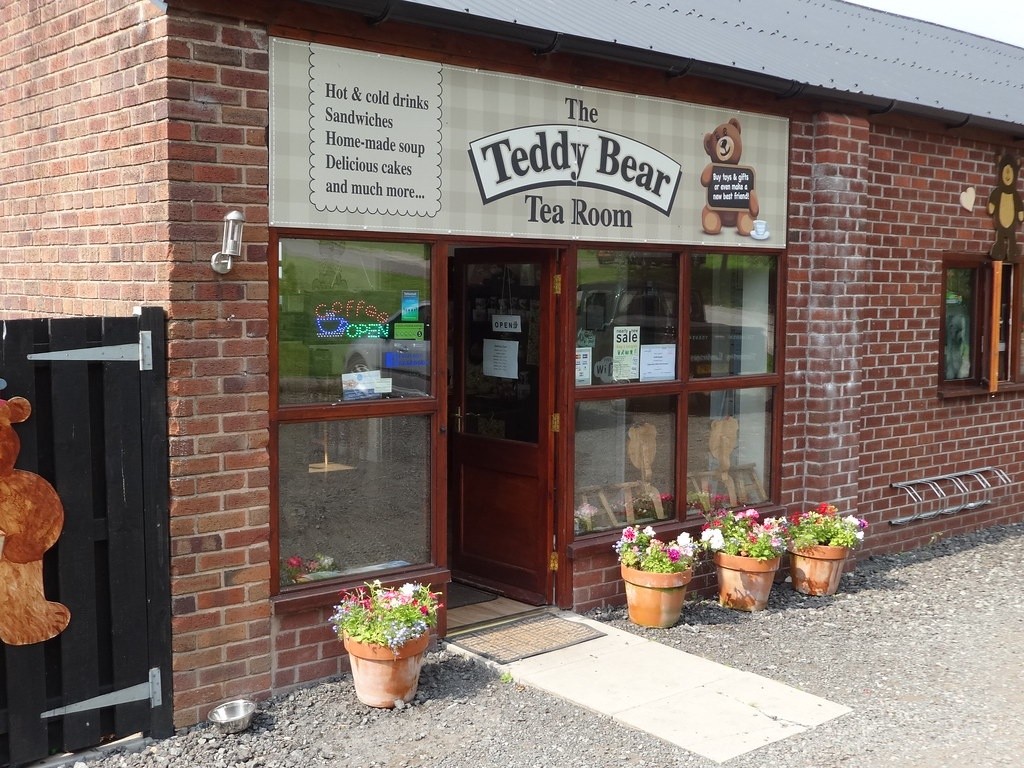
top-left (211, 210), bottom-right (246, 274)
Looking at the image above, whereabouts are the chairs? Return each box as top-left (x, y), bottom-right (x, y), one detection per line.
top-left (580, 423), bottom-right (665, 532)
top-left (687, 416), bottom-right (769, 509)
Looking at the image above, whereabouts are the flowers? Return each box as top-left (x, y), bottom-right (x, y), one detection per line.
top-left (611, 526), bottom-right (703, 573)
top-left (574, 503), bottom-right (598, 521)
top-left (687, 490), bottom-right (730, 511)
top-left (610, 494), bottom-right (673, 512)
top-left (278, 551), bottom-right (335, 586)
top-left (699, 509), bottom-right (787, 561)
top-left (328, 579), bottom-right (444, 661)
top-left (784, 502), bottom-right (868, 552)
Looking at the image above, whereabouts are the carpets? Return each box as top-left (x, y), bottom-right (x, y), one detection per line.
top-left (446, 582), bottom-right (498, 609)
top-left (441, 612), bottom-right (608, 665)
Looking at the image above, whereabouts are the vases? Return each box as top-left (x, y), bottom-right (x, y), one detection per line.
top-left (788, 540), bottom-right (849, 596)
top-left (342, 630), bottom-right (428, 707)
top-left (712, 551), bottom-right (780, 611)
top-left (621, 562), bottom-right (691, 629)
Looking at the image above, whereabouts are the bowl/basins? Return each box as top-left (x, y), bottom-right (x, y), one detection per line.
top-left (208, 700), bottom-right (257, 733)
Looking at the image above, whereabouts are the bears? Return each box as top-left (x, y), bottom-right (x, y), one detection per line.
top-left (944, 315), bottom-right (970, 380)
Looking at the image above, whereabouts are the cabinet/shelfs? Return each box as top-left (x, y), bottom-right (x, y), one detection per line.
top-left (448, 263), bottom-right (539, 442)
